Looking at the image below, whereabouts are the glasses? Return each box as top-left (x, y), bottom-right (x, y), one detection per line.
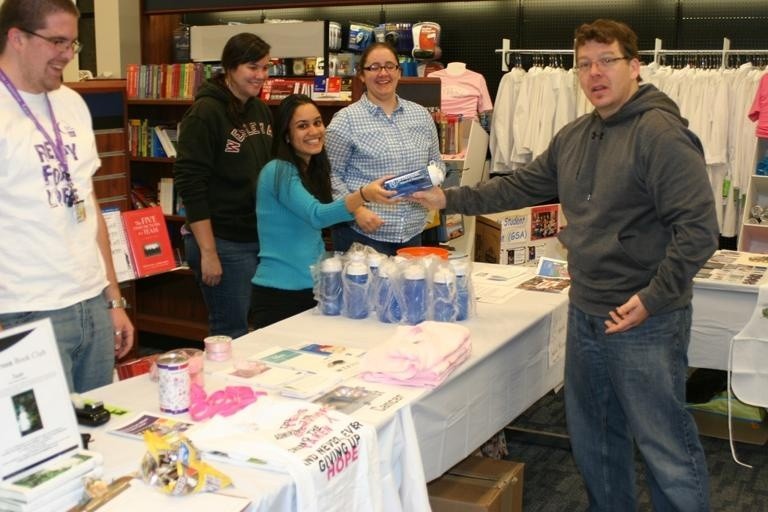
top-left (18, 28), bottom-right (84, 53)
top-left (578, 57), bottom-right (628, 72)
top-left (364, 64), bottom-right (400, 72)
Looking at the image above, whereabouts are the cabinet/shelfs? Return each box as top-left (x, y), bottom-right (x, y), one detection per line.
top-left (62, 79), bottom-right (141, 365)
top-left (735, 134), bottom-right (768, 255)
top-left (126, 77), bottom-right (441, 345)
top-left (439, 118), bottom-right (490, 264)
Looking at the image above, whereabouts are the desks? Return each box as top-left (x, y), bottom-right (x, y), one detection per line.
top-left (688, 250), bottom-right (768, 410)
top-left (0, 264), bottom-right (569, 512)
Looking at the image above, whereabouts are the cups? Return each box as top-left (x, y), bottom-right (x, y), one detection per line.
top-left (163, 348), bottom-right (205, 375)
top-left (203, 334), bottom-right (233, 361)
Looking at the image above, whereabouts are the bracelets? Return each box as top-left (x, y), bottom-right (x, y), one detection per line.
top-left (358, 186), bottom-right (372, 204)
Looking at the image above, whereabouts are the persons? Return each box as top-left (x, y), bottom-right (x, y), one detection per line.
top-left (248, 92), bottom-right (407, 332)
top-left (322, 41), bottom-right (447, 257)
top-left (425, 61), bottom-right (494, 120)
top-left (172, 33), bottom-right (278, 340)
top-left (408, 19), bottom-right (721, 511)
top-left (0, 0), bottom-right (135, 393)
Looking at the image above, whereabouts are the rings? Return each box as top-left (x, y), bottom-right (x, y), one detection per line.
top-left (114, 331), bottom-right (123, 336)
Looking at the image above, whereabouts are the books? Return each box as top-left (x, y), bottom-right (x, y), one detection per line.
top-left (108, 411), bottom-right (197, 446)
top-left (436, 212), bottom-right (465, 242)
top-left (101, 61), bottom-right (227, 284)
top-left (1, 449), bottom-right (104, 512)
top-left (691, 248), bottom-right (768, 290)
top-left (514, 276), bottom-right (571, 294)
top-left (535, 256), bottom-right (571, 281)
top-left (429, 112), bottom-right (465, 154)
top-left (256, 51), bottom-right (358, 101)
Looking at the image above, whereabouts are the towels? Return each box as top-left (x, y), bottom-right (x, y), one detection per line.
top-left (362, 320), bottom-right (472, 390)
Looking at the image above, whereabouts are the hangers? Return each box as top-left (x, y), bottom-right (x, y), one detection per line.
top-left (506, 49), bottom-right (768, 72)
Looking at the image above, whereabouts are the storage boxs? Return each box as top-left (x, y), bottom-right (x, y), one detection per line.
top-left (424, 454), bottom-right (526, 512)
top-left (474, 202), bottom-right (568, 265)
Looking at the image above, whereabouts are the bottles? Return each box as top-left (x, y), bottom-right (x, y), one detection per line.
top-left (316, 247), bottom-right (471, 327)
top-left (382, 160), bottom-right (446, 200)
top-left (439, 121), bottom-right (449, 155)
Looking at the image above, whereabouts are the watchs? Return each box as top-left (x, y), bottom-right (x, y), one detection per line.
top-left (105, 296), bottom-right (128, 310)
